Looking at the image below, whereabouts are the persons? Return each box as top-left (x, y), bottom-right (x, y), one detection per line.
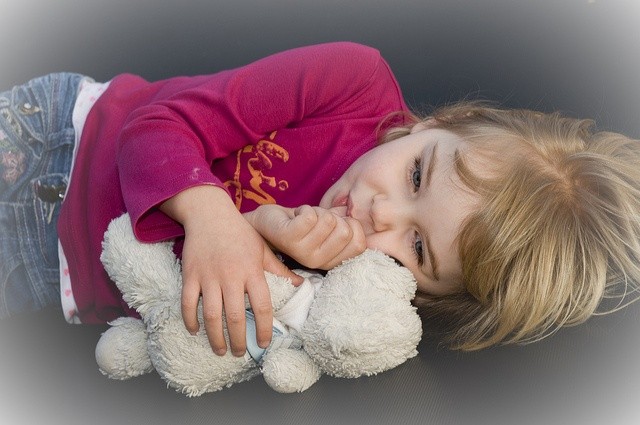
top-left (0, 41), bottom-right (640, 359)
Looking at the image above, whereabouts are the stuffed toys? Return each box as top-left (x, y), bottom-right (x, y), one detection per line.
top-left (94, 211), bottom-right (423, 399)
